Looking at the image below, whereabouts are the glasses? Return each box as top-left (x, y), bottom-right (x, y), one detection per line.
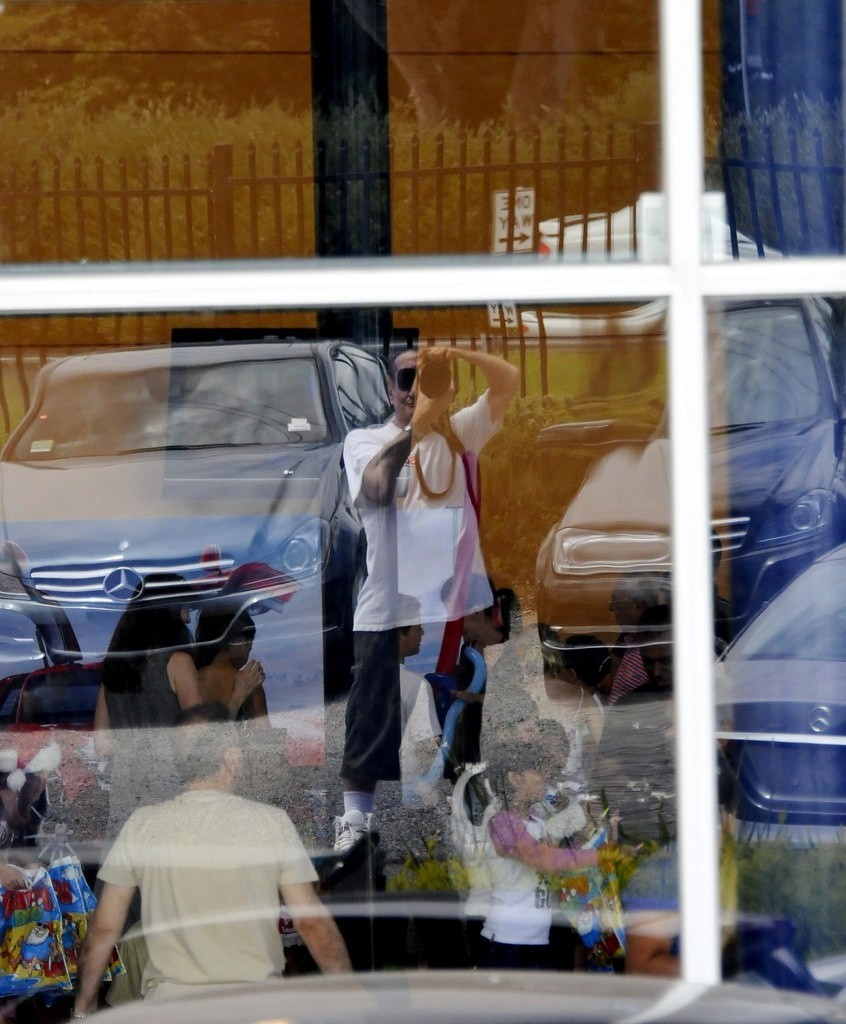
top-left (609, 598), bottom-right (629, 607)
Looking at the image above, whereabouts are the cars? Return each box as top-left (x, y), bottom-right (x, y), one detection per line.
top-left (2, 210), bottom-right (846, 1024)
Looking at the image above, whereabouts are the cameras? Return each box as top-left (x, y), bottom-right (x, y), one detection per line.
top-left (412, 356), bottom-right (454, 399)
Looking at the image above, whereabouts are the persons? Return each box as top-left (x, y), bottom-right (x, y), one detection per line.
top-left (335, 343), bottom-right (523, 859)
top-left (64, 571), bottom-right (684, 1024)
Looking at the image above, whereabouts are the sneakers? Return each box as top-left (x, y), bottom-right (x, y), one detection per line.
top-left (446, 795), bottom-right (494, 858)
top-left (333, 809), bottom-right (370, 869)
